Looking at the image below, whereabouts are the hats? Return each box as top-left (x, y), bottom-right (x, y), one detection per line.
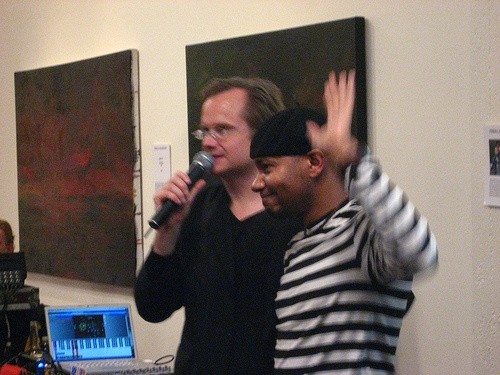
top-left (250, 105), bottom-right (328, 159)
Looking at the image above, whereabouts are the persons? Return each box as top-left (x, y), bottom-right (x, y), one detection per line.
top-left (251, 69), bottom-right (439, 375)
top-left (134, 75), bottom-right (303, 375)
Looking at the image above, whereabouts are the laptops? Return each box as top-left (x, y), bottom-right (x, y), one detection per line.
top-left (44, 303), bottom-right (172, 375)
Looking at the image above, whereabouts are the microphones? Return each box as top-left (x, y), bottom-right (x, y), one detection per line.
top-left (148, 151), bottom-right (215, 229)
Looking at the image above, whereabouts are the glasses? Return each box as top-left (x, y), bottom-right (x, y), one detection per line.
top-left (191, 125), bottom-right (246, 140)
